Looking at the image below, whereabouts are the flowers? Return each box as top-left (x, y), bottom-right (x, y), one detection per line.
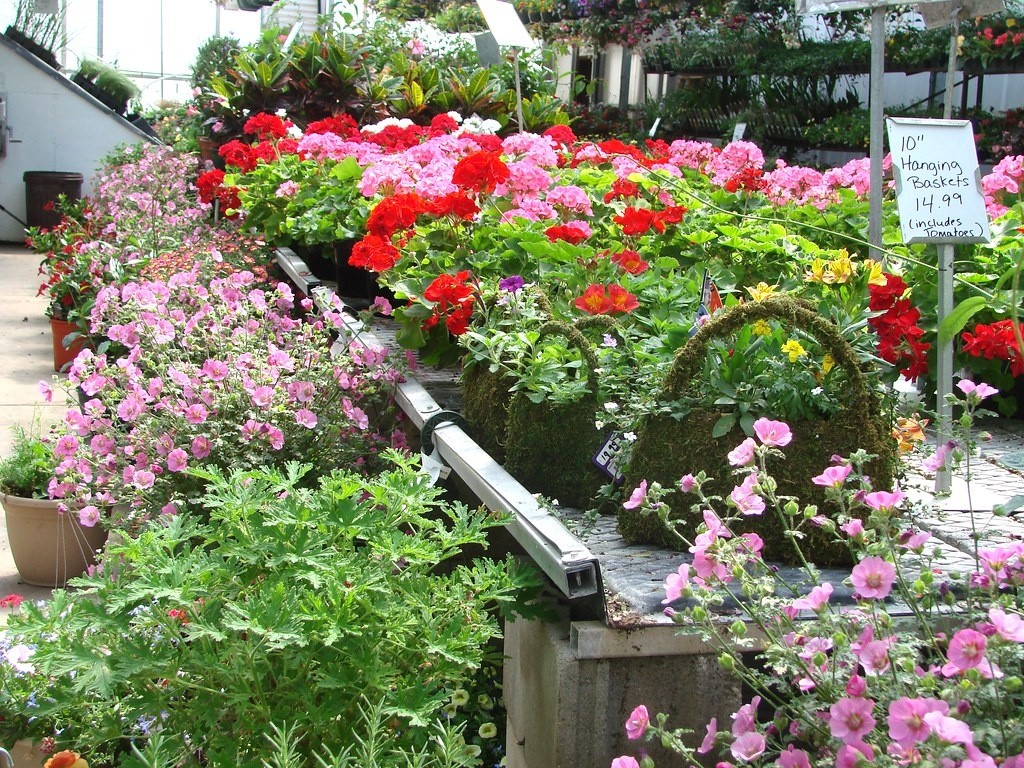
top-left (0, 0), bottom-right (1024, 768)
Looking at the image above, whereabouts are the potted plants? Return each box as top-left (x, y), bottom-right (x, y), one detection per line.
top-left (4, 0), bottom-right (74, 72)
top-left (0, 416), bottom-right (108, 589)
top-left (71, 53), bottom-right (143, 118)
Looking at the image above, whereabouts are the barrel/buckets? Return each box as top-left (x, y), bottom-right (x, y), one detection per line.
top-left (24, 172), bottom-right (83, 230)
top-left (49, 319), bottom-right (90, 373)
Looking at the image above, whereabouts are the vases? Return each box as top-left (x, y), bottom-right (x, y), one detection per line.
top-left (48, 317), bottom-right (97, 373)
top-left (307, 241), bottom-right (342, 284)
top-left (367, 272), bottom-right (408, 318)
top-left (330, 238), bottom-right (368, 299)
top-left (197, 136), bottom-right (220, 164)
